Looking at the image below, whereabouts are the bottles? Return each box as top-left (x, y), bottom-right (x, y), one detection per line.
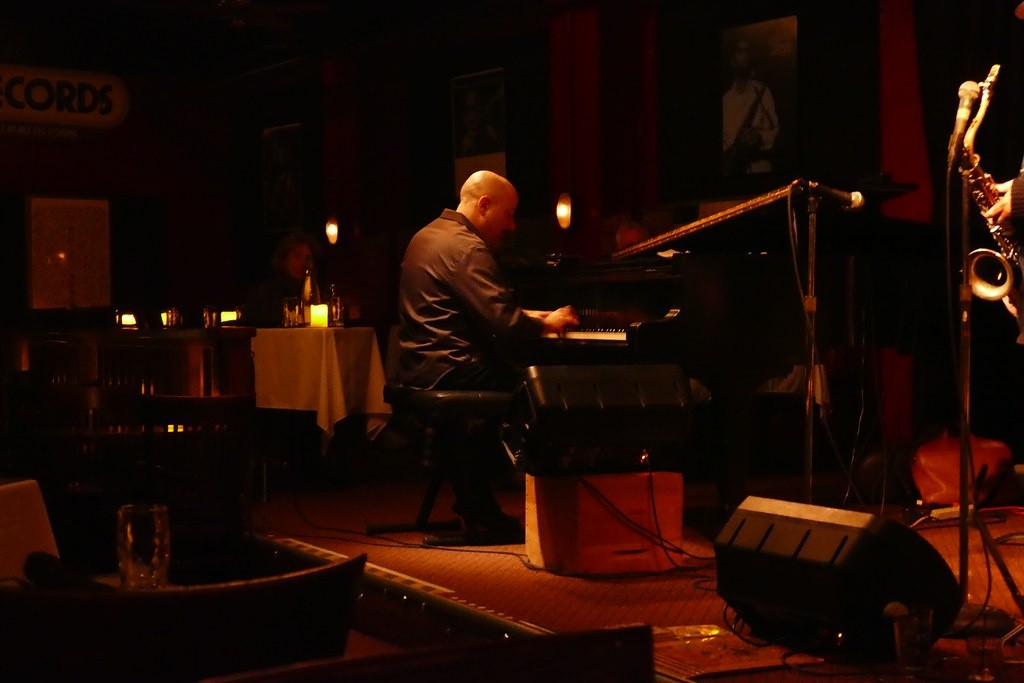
top-left (301, 256), bottom-right (321, 327)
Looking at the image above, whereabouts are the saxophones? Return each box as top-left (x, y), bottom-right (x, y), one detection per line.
top-left (956, 61), bottom-right (1024, 347)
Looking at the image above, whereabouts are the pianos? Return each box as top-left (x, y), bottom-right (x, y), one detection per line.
top-left (521, 248), bottom-right (741, 505)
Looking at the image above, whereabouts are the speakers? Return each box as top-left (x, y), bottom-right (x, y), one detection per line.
top-left (713, 496), bottom-right (964, 663)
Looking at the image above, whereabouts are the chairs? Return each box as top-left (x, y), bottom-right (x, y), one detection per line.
top-left (0, 553), bottom-right (366, 683)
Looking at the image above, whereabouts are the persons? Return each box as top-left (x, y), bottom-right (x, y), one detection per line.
top-left (981, 1), bottom-right (1023, 461)
top-left (241, 234), bottom-right (365, 494)
top-left (721, 33), bottom-right (785, 196)
top-left (386, 167), bottom-right (583, 530)
top-left (453, 87), bottom-right (507, 155)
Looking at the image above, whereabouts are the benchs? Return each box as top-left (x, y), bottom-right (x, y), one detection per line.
top-left (365, 383), bottom-right (527, 548)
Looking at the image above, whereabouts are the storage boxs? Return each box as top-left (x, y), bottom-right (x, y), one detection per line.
top-left (525, 471), bottom-right (684, 577)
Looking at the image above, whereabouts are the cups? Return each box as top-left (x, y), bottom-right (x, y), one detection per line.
top-left (283, 296), bottom-right (302, 327)
top-left (327, 296), bottom-right (345, 327)
top-left (116, 503), bottom-right (170, 593)
top-left (203, 305), bottom-right (217, 328)
top-left (167, 308), bottom-right (182, 328)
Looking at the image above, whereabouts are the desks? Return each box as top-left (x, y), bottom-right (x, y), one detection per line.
top-left (249, 327), bottom-right (393, 492)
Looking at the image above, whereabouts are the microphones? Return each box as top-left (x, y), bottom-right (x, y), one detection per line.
top-left (799, 178), bottom-right (865, 210)
top-left (948, 80), bottom-right (981, 168)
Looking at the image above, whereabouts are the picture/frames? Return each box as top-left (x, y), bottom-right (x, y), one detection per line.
top-left (451, 67), bottom-right (507, 197)
top-left (262, 123), bottom-right (305, 233)
